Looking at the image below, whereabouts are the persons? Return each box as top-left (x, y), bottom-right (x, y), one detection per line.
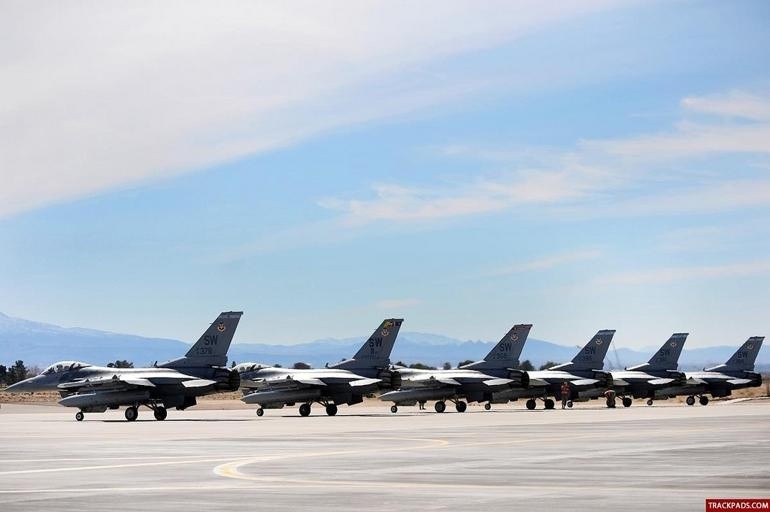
top-left (600, 390), bottom-right (617, 406)
top-left (419, 403), bottom-right (425, 411)
top-left (561, 382), bottom-right (570, 409)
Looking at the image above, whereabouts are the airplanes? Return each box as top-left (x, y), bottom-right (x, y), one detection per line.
top-left (4, 311), bottom-right (765, 421)
